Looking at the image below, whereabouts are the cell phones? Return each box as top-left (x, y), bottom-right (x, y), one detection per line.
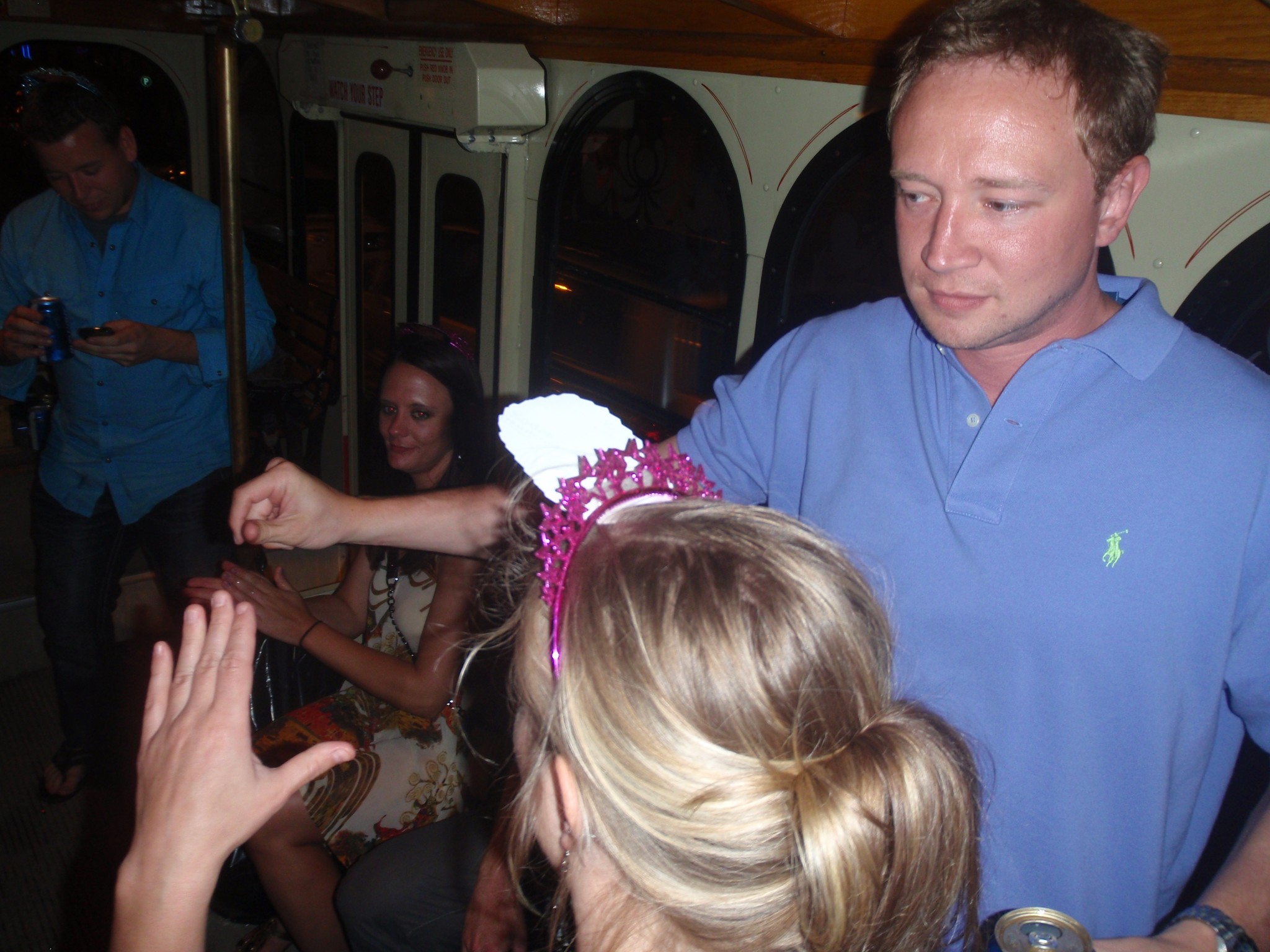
top-left (76, 326), bottom-right (113, 343)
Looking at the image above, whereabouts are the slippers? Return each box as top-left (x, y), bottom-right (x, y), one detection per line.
top-left (39, 753), bottom-right (96, 805)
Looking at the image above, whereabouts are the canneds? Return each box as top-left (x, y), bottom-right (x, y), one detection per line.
top-left (979, 907), bottom-right (1095, 952)
top-left (30, 296), bottom-right (73, 365)
top-left (29, 394), bottom-right (53, 450)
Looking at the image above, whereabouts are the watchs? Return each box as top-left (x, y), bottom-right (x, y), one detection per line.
top-left (1164, 904), bottom-right (1260, 952)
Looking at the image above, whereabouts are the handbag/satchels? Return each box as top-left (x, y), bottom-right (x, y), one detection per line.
top-left (248, 632), bottom-right (345, 730)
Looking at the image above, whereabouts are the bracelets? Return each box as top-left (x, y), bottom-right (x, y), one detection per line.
top-left (299, 620), bottom-right (323, 649)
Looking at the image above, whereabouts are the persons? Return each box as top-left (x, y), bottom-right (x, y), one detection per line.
top-left (183, 337), bottom-right (500, 952)
top-left (0, 92), bottom-right (277, 804)
top-left (227, 0), bottom-right (1270, 952)
top-left (110, 437), bottom-right (977, 952)
